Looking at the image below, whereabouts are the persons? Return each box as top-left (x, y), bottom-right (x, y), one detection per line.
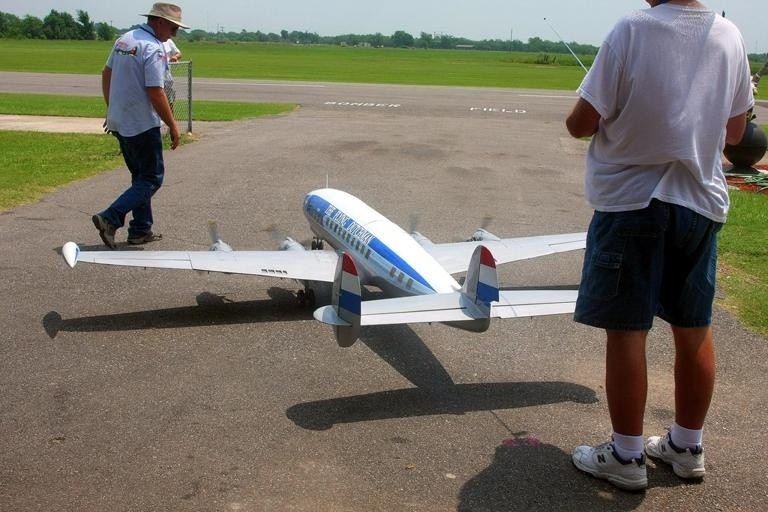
top-left (563, 0), bottom-right (756, 491)
top-left (162, 36), bottom-right (183, 119)
top-left (90, 1), bottom-right (191, 251)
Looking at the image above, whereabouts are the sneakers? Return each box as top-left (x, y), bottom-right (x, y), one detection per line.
top-left (571, 440), bottom-right (649, 490)
top-left (645, 434), bottom-right (706, 479)
top-left (125, 232), bottom-right (163, 246)
top-left (91, 213), bottom-right (118, 251)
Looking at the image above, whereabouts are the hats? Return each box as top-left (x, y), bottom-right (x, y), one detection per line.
top-left (138, 2), bottom-right (190, 32)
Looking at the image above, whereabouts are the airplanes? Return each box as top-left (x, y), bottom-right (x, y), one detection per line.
top-left (61, 175), bottom-right (588, 328)
